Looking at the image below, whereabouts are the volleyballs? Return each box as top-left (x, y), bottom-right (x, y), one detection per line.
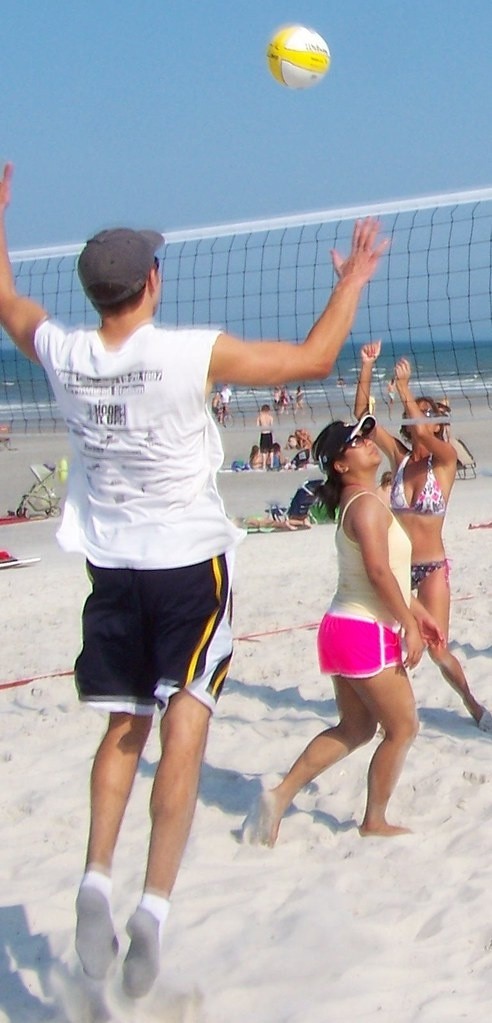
top-left (266, 24), bottom-right (332, 89)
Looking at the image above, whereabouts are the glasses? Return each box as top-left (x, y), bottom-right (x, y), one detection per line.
top-left (340, 432), bottom-right (370, 455)
top-left (152, 256), bottom-right (160, 271)
top-left (422, 409), bottom-right (437, 417)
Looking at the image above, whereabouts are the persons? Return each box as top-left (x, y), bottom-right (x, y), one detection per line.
top-left (0, 162), bottom-right (392, 996)
top-left (352, 339), bottom-right (492, 734)
top-left (227, 513), bottom-right (312, 532)
top-left (270, 383), bottom-right (305, 413)
top-left (249, 403), bottom-right (313, 473)
top-left (369, 394), bottom-right (376, 416)
top-left (212, 384), bottom-right (232, 425)
top-left (388, 376), bottom-right (396, 421)
top-left (375, 472), bottom-right (394, 510)
top-left (337, 378), bottom-right (345, 385)
top-left (250, 413), bottom-right (447, 849)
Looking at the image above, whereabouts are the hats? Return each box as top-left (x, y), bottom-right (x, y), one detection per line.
top-left (437, 400), bottom-right (451, 412)
top-left (78, 228), bottom-right (165, 305)
top-left (314, 414), bottom-right (377, 475)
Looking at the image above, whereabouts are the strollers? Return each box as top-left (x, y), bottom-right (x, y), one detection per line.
top-left (15, 459), bottom-right (70, 519)
